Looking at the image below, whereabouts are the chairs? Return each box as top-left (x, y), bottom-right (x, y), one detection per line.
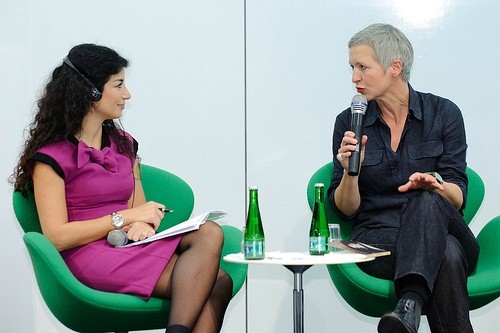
top-left (12, 164), bottom-right (248, 333)
top-left (307, 157), bottom-right (500, 318)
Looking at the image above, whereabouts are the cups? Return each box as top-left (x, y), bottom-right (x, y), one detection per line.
top-left (327, 224), bottom-right (341, 252)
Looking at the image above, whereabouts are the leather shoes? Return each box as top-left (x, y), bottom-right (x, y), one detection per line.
top-left (378, 299), bottom-right (422, 333)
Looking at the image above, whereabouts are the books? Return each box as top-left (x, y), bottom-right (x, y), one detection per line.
top-left (324, 239), bottom-right (391, 259)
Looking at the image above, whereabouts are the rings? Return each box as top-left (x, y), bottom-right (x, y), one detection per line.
top-left (143, 232), bottom-right (147, 237)
top-left (340, 153), bottom-right (345, 159)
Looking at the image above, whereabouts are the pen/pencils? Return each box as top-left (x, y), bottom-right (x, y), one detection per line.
top-left (158, 208), bottom-right (174, 213)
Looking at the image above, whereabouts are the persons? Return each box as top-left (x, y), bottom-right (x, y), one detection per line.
top-left (327, 22), bottom-right (482, 333)
top-left (13, 43), bottom-right (234, 333)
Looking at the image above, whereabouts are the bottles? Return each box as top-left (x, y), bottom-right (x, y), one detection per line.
top-left (309, 182), bottom-right (330, 255)
top-left (243, 187), bottom-right (266, 260)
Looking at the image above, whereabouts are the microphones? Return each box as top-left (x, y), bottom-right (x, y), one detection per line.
top-left (348, 95), bottom-right (367, 175)
top-left (107, 229), bottom-right (134, 247)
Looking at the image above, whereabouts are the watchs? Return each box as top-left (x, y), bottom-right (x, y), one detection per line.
top-left (425, 171), bottom-right (444, 185)
top-left (110, 210), bottom-right (125, 229)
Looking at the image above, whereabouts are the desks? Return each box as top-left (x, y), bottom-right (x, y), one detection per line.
top-left (223, 248), bottom-right (376, 333)
